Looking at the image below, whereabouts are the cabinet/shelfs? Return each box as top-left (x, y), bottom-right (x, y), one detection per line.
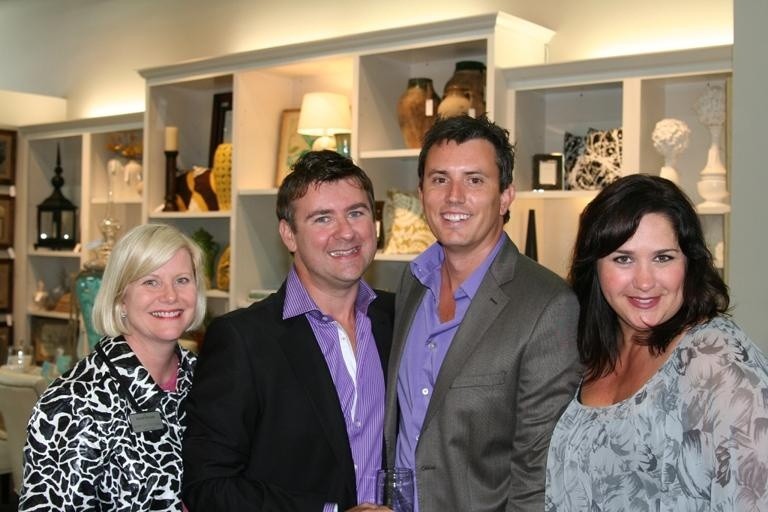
top-left (1, 9), bottom-right (732, 364)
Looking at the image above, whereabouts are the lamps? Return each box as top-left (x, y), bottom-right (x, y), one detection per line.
top-left (299, 90), bottom-right (351, 153)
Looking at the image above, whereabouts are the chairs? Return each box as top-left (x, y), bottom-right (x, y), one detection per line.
top-left (2, 375), bottom-right (49, 495)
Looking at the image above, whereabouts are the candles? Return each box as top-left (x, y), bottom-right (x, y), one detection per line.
top-left (163, 121), bottom-right (178, 153)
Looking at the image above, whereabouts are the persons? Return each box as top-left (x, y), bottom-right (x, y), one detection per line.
top-left (180, 150), bottom-right (398, 511)
top-left (545, 174), bottom-right (765, 510)
top-left (20, 225), bottom-right (206, 512)
top-left (382, 116), bottom-right (579, 510)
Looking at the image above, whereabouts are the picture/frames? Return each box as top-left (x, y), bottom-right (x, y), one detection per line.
top-left (0, 127), bottom-right (18, 189)
top-left (533, 153), bottom-right (561, 186)
top-left (0, 258), bottom-right (15, 315)
top-left (0, 193), bottom-right (15, 251)
top-left (274, 108), bottom-right (304, 186)
